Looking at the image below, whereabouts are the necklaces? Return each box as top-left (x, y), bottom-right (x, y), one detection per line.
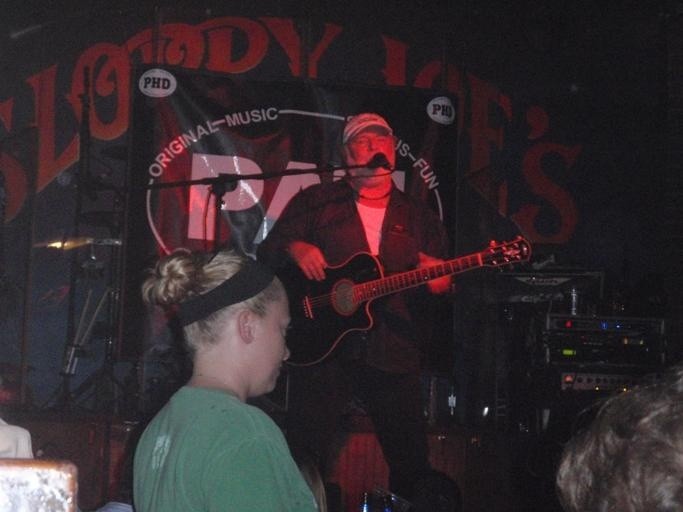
top-left (359, 191), bottom-right (391, 201)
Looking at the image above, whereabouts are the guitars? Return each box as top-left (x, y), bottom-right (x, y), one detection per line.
top-left (282, 237), bottom-right (531, 366)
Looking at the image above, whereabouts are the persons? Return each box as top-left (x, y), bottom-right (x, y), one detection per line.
top-left (285, 432), bottom-right (327, 512)
top-left (133, 248), bottom-right (320, 512)
top-left (554, 364), bottom-right (683, 512)
top-left (256, 111), bottom-right (453, 512)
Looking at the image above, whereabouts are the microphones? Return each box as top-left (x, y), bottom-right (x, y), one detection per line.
top-left (373, 152), bottom-right (396, 174)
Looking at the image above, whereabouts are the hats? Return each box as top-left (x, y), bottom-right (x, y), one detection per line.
top-left (343, 113), bottom-right (392, 145)
